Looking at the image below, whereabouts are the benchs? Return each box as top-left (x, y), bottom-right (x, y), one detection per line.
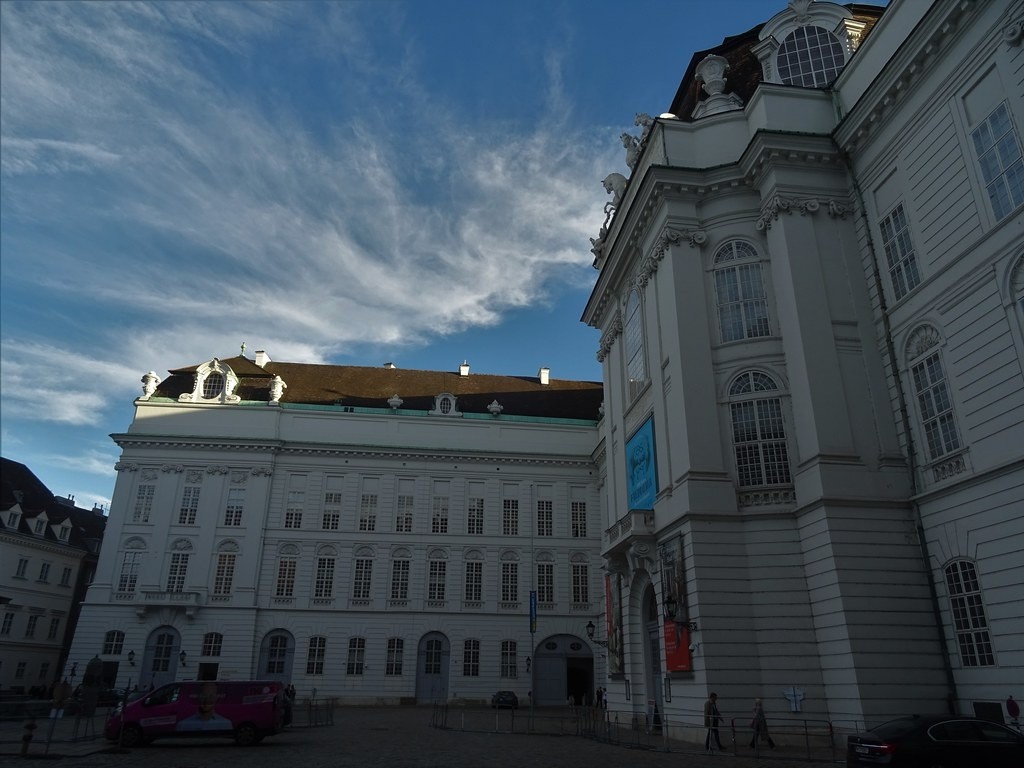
top-left (447, 697), bottom-right (489, 708)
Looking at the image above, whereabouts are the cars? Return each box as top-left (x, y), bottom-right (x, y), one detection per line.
top-left (490, 691), bottom-right (518, 709)
top-left (848, 714), bottom-right (1024, 768)
top-left (97, 689), bottom-right (127, 708)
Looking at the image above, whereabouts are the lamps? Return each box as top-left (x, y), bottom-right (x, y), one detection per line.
top-left (586, 620), bottom-right (607, 646)
top-left (526, 657), bottom-right (532, 673)
top-left (664, 595), bottom-right (697, 630)
top-left (180, 650), bottom-right (186, 666)
top-left (128, 649), bottom-right (135, 666)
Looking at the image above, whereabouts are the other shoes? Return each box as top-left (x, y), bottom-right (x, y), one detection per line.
top-left (719, 746), bottom-right (726, 750)
top-left (705, 747), bottom-right (712, 750)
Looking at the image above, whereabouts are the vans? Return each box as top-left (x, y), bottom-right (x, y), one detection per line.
top-left (104, 679), bottom-right (284, 748)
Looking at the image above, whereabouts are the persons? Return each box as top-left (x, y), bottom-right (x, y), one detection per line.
top-left (176, 683), bottom-right (233, 730)
top-left (602, 688), bottom-right (607, 709)
top-left (283, 684), bottom-right (297, 705)
top-left (748, 699), bottom-right (779, 751)
top-left (595, 686), bottom-right (603, 709)
top-left (703, 693), bottom-right (728, 751)
